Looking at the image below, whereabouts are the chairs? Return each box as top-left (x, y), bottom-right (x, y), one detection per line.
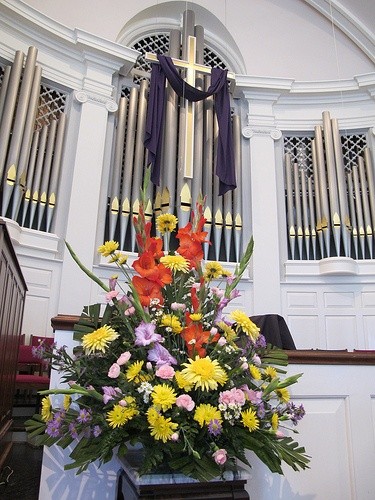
top-left (13, 345), bottom-right (50, 418)
top-left (29, 333), bottom-right (56, 377)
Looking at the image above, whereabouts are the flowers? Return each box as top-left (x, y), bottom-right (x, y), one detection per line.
top-left (18, 163), bottom-right (315, 484)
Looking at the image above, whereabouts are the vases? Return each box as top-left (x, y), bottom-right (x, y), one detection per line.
top-left (112, 447), bottom-right (254, 500)
